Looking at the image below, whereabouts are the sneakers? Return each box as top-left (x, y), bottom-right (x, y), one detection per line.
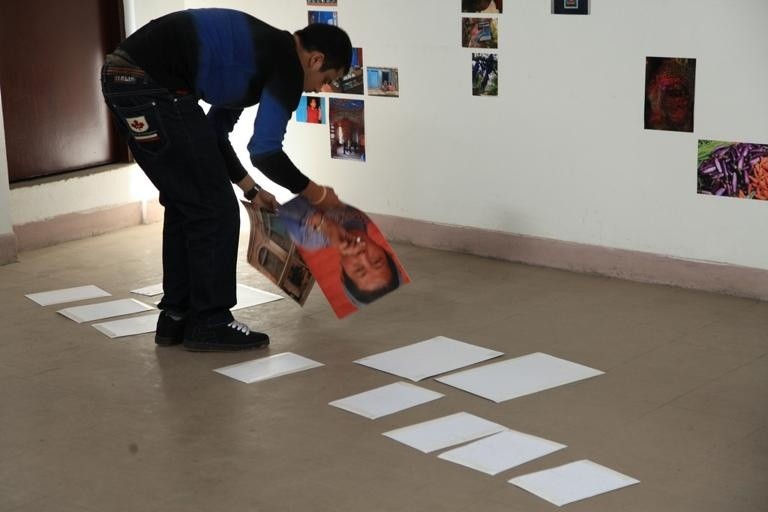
top-left (184, 319), bottom-right (270, 351)
top-left (154, 310), bottom-right (186, 348)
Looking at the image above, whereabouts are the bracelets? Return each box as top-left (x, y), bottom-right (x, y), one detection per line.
top-left (309, 187), bottom-right (326, 205)
top-left (241, 185), bottom-right (261, 201)
top-left (316, 213), bottom-right (326, 232)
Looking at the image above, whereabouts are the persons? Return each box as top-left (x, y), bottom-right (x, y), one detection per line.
top-left (100, 5), bottom-right (353, 352)
top-left (306, 96), bottom-right (322, 123)
top-left (274, 196), bottom-right (399, 304)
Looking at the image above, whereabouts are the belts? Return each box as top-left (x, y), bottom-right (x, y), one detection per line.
top-left (101, 64), bottom-right (146, 79)
